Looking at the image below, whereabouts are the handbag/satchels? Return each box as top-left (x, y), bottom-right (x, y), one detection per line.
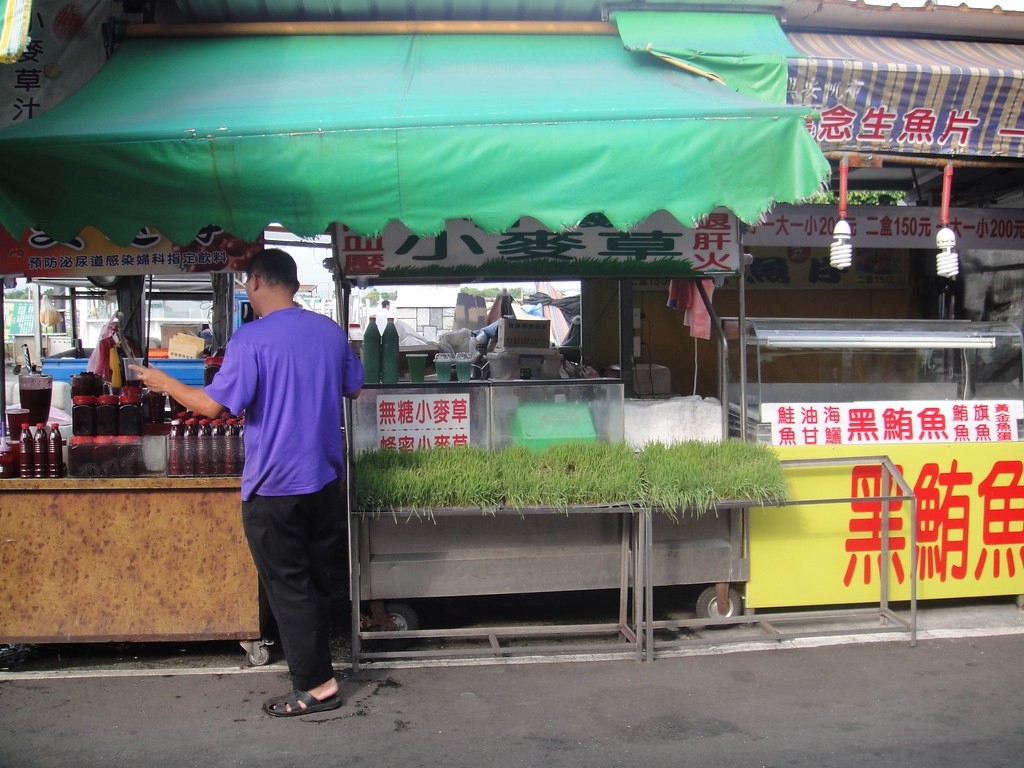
top-left (110, 341), bottom-right (123, 388)
top-left (95, 325), bottom-right (119, 382)
top-left (87, 310), bottom-right (118, 374)
top-left (39, 295), bottom-right (63, 326)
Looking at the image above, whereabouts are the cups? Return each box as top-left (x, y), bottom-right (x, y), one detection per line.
top-left (406, 354), bottom-right (428, 384)
top-left (455, 352), bottom-right (473, 383)
top-left (17, 375), bottom-right (54, 426)
top-left (433, 353), bottom-right (453, 382)
top-left (7, 408), bottom-right (30, 442)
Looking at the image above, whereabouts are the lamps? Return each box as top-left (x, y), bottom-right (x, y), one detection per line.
top-left (766, 337), bottom-right (996, 349)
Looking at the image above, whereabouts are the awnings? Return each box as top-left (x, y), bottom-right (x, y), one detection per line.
top-left (0, 8), bottom-right (836, 250)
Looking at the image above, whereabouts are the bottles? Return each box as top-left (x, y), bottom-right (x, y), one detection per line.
top-left (47, 423), bottom-right (63, 478)
top-left (362, 316), bottom-right (382, 384)
top-left (71, 386), bottom-right (165, 477)
top-left (19, 423), bottom-right (34, 478)
top-left (349, 324), bottom-right (363, 341)
top-left (33, 423), bottom-right (48, 477)
top-left (169, 410), bottom-right (246, 439)
top-left (381, 318), bottom-right (400, 384)
top-left (204, 356), bottom-right (223, 390)
top-left (0, 437), bottom-right (16, 478)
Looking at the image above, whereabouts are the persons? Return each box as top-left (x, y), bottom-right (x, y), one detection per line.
top-left (128, 247), bottom-right (366, 717)
top-left (376, 300), bottom-right (396, 336)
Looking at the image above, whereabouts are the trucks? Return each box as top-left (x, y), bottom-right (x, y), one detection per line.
top-left (33, 273), bottom-right (314, 407)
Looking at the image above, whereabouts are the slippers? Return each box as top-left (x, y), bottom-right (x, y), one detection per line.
top-left (264, 690), bottom-right (340, 717)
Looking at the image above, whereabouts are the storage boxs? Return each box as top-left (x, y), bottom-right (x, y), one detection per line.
top-left (160, 323), bottom-right (203, 348)
top-left (168, 333), bottom-right (205, 358)
top-left (498, 316), bottom-right (551, 349)
top-left (486, 352), bottom-right (562, 379)
